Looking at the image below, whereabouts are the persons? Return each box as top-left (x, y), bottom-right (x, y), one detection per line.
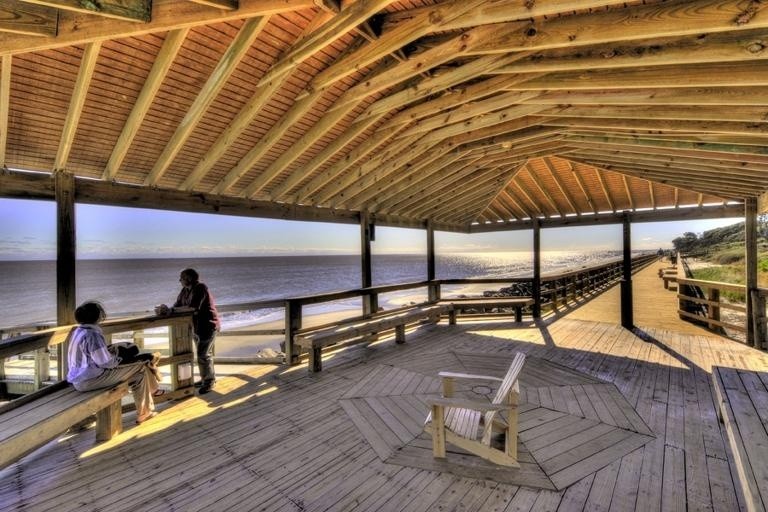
top-left (658, 248), bottom-right (677, 264)
top-left (64, 299), bottom-right (166, 425)
top-left (154, 268), bottom-right (221, 395)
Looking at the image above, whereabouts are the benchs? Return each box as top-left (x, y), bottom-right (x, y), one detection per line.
top-left (0, 379), bottom-right (129, 470)
top-left (711, 364), bottom-right (768, 511)
top-left (293, 295), bottom-right (535, 372)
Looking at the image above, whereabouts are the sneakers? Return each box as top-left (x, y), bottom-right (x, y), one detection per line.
top-left (195, 380), bottom-right (216, 393)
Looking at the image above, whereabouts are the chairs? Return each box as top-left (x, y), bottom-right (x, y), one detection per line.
top-left (421, 351), bottom-right (528, 470)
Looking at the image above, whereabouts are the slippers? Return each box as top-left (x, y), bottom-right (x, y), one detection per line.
top-left (152, 390), bottom-right (165, 397)
top-left (136, 411), bottom-right (159, 423)
